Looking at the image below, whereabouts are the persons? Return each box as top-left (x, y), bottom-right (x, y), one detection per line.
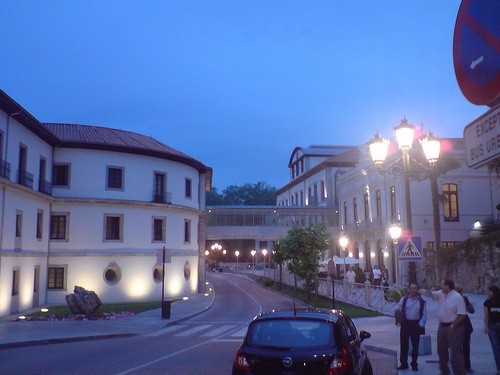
top-left (483, 286), bottom-right (500, 375)
top-left (347, 267), bottom-right (356, 295)
top-left (372, 264), bottom-right (390, 300)
top-left (428, 278), bottom-right (475, 375)
top-left (396, 282), bottom-right (426, 371)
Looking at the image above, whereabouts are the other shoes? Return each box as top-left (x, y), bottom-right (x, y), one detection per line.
top-left (413, 366), bottom-right (418, 371)
top-left (397, 366), bottom-right (408, 370)
top-left (467, 368), bottom-right (473, 374)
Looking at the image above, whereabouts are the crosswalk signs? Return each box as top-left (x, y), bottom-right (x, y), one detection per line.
top-left (397, 235), bottom-right (424, 262)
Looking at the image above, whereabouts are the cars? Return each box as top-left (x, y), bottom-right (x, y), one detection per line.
top-left (231, 306), bottom-right (374, 375)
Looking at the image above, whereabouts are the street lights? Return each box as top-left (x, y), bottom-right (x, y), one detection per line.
top-left (368, 115), bottom-right (441, 291)
top-left (338, 236), bottom-right (349, 301)
top-left (388, 222), bottom-right (402, 285)
top-left (205, 243), bottom-right (229, 274)
top-left (251, 249), bottom-right (257, 274)
top-left (235, 250), bottom-right (240, 273)
top-left (261, 249), bottom-right (268, 278)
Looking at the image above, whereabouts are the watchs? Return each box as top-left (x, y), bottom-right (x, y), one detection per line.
top-left (451, 324), bottom-right (454, 327)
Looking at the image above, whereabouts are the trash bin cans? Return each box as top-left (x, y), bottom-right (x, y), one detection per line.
top-left (161, 300), bottom-right (171, 319)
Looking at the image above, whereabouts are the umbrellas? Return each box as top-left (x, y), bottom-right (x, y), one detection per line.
top-left (320, 255), bottom-right (364, 265)
